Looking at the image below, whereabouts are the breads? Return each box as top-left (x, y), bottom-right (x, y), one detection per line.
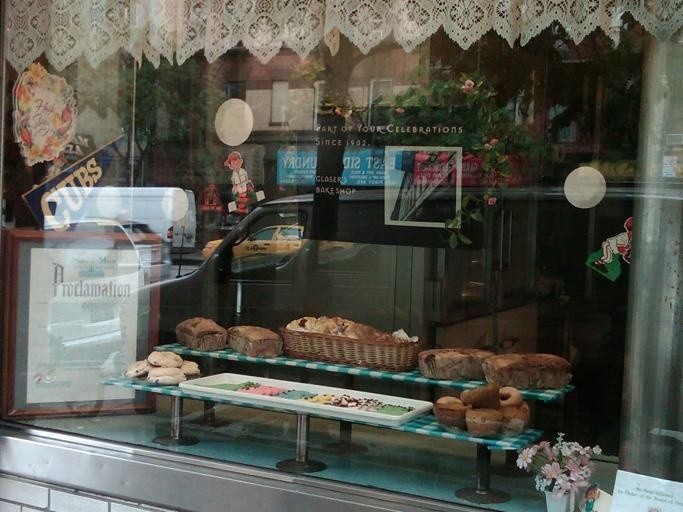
top-left (227, 325), bottom-right (284, 358)
top-left (175, 316), bottom-right (227, 351)
top-left (125, 351), bottom-right (202, 385)
top-left (286, 315), bottom-right (411, 345)
top-left (418, 347), bottom-right (496, 380)
top-left (433, 382), bottom-right (530, 437)
top-left (482, 352), bottom-right (574, 389)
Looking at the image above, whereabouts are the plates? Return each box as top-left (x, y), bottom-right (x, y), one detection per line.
top-left (179, 371), bottom-right (432, 431)
top-left (563, 167), bottom-right (607, 208)
top-left (213, 99), bottom-right (253, 148)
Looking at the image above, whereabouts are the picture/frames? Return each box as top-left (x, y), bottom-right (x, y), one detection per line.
top-left (382, 145), bottom-right (462, 232)
top-left (0, 228), bottom-right (164, 422)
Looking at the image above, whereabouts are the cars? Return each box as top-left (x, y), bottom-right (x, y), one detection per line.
top-left (200, 224), bottom-right (369, 272)
top-left (37, 216), bottom-right (170, 353)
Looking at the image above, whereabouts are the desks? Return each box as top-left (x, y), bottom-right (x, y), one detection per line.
top-left (99, 341), bottom-right (574, 507)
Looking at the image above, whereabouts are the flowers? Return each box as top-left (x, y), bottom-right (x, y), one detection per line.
top-left (379, 61), bottom-right (541, 253)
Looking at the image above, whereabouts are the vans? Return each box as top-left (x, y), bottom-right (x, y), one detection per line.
top-left (43, 186), bottom-right (198, 249)
top-left (119, 179), bottom-right (683, 438)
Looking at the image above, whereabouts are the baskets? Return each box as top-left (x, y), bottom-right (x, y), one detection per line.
top-left (281, 325), bottom-right (417, 369)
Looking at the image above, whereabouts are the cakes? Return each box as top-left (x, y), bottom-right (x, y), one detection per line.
top-left (17, 63), bottom-right (78, 167)
